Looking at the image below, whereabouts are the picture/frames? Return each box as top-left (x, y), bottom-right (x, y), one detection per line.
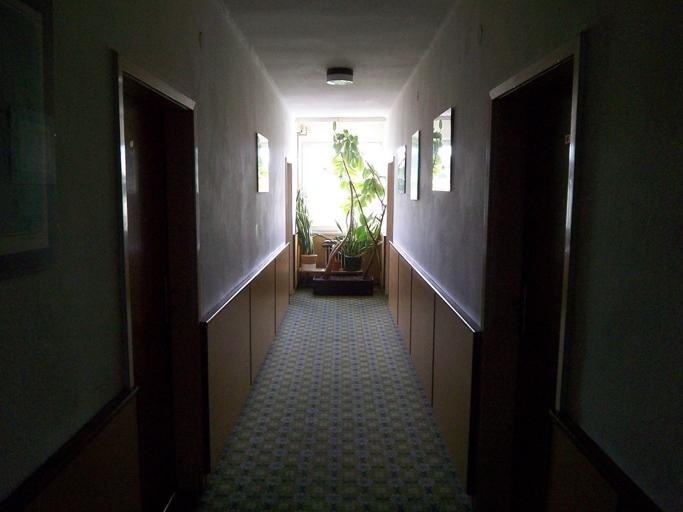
top-left (0, 0), bottom-right (56, 278)
top-left (257, 132), bottom-right (270, 192)
top-left (397, 105), bottom-right (452, 201)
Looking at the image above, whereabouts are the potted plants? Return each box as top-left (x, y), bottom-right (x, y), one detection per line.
top-left (295, 190), bottom-right (318, 270)
top-left (330, 216), bottom-right (379, 269)
